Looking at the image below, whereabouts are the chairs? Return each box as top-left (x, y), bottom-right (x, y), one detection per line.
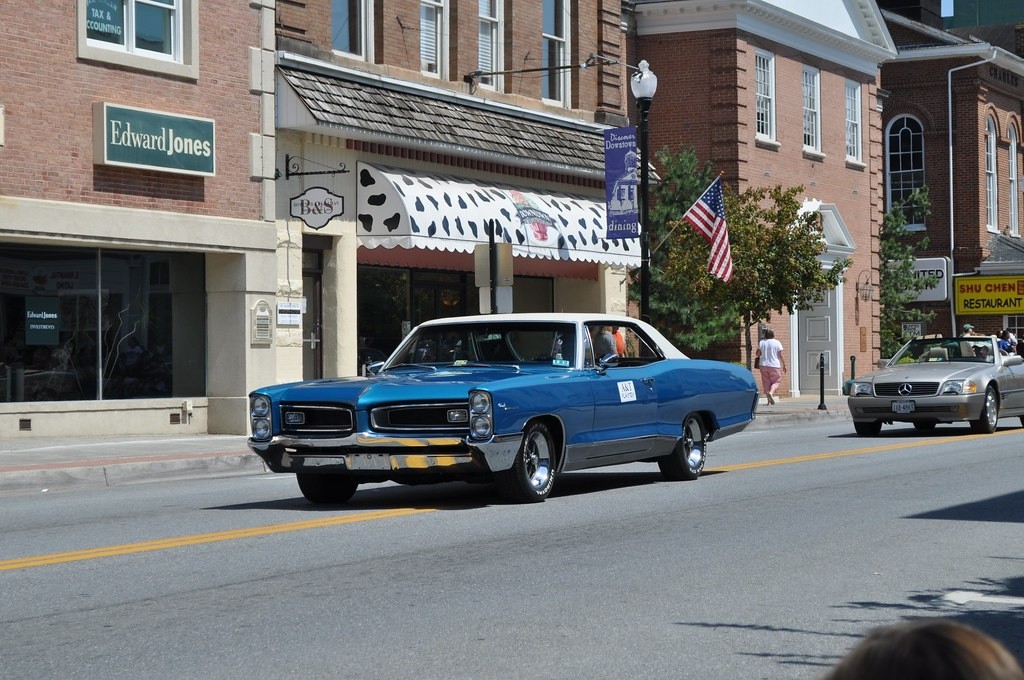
top-left (927, 347), bottom-right (948, 362)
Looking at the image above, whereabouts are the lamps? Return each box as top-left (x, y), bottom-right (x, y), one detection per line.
top-left (857, 270), bottom-right (872, 303)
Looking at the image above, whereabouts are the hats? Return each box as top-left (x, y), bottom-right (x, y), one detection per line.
top-left (972, 342), bottom-right (990, 350)
top-left (963, 324), bottom-right (974, 332)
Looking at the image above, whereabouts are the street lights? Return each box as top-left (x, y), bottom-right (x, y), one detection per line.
top-left (631, 58), bottom-right (658, 357)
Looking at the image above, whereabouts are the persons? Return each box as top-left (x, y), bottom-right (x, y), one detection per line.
top-left (593, 325), bottom-right (618, 360)
top-left (997, 328), bottom-right (1024, 357)
top-left (755, 328), bottom-right (787, 406)
top-left (612, 324), bottom-right (625, 357)
top-left (51, 339), bottom-right (75, 372)
top-left (584, 325), bottom-right (595, 360)
top-left (959, 323), bottom-right (978, 358)
top-left (123, 336), bottom-right (143, 375)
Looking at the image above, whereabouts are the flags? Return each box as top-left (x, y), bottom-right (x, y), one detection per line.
top-left (684, 180), bottom-right (733, 284)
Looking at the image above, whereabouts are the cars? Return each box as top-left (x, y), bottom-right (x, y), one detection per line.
top-left (847, 334), bottom-right (1024, 432)
top-left (245, 313), bottom-right (758, 501)
